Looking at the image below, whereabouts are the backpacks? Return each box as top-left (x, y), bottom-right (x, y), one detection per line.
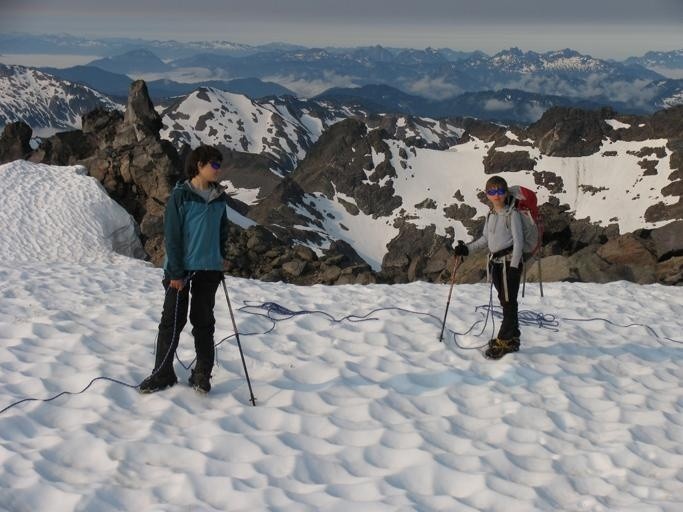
top-left (504, 185), bottom-right (544, 264)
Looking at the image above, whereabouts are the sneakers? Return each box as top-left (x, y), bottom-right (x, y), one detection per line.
top-left (137, 366), bottom-right (178, 393)
top-left (187, 367), bottom-right (212, 394)
top-left (485, 337), bottom-right (521, 360)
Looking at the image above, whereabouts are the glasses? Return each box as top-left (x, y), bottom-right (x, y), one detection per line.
top-left (208, 159), bottom-right (221, 169)
top-left (486, 188), bottom-right (507, 196)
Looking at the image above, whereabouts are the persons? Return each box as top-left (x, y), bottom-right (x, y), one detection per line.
top-left (131, 144), bottom-right (231, 394)
top-left (453, 176), bottom-right (524, 361)
